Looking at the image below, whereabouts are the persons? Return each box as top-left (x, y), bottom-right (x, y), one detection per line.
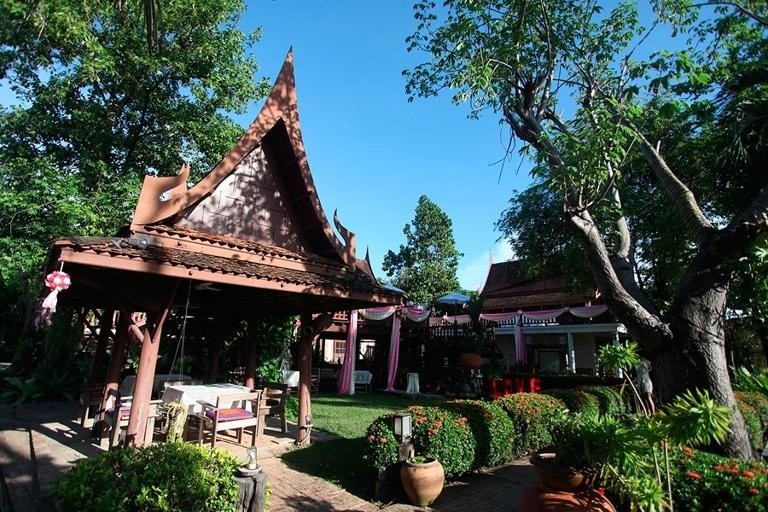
top-left (635, 357), bottom-right (656, 417)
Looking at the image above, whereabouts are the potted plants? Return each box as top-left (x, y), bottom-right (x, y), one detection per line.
top-left (402, 457), bottom-right (444, 505)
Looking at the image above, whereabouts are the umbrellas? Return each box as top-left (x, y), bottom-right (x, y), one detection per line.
top-left (438, 291), bottom-right (473, 323)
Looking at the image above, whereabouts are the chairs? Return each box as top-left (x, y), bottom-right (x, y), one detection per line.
top-left (196, 393), bottom-right (262, 449)
top-left (98, 383), bottom-right (163, 451)
top-left (310, 366), bottom-right (372, 395)
top-left (250, 380), bottom-right (288, 433)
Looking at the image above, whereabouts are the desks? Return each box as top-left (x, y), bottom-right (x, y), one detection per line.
top-left (277, 369), bottom-right (300, 395)
top-left (120, 374), bottom-right (192, 404)
top-left (163, 383), bottom-right (252, 412)
